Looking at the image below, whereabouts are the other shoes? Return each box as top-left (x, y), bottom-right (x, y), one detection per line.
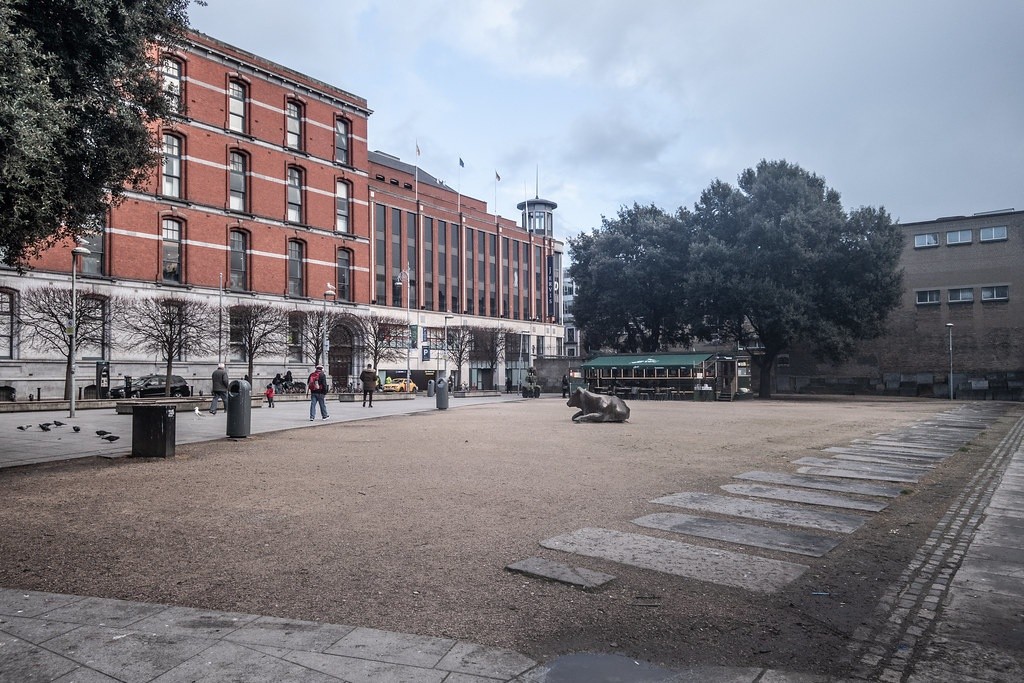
top-left (310, 416), bottom-right (314, 420)
top-left (322, 414), bottom-right (331, 421)
top-left (209, 411), bottom-right (215, 414)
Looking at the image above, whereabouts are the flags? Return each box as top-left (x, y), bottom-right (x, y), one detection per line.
top-left (496, 173), bottom-right (500, 181)
top-left (460, 159), bottom-right (464, 167)
top-left (417, 146), bottom-right (420, 156)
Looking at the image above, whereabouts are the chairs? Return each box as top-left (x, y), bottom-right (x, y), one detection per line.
top-left (593, 387), bottom-right (685, 400)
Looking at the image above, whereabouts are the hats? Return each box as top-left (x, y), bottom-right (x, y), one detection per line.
top-left (218, 363), bottom-right (225, 368)
top-left (315, 365), bottom-right (324, 370)
top-left (267, 384), bottom-right (272, 388)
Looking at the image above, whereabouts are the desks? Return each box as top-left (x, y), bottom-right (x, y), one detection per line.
top-left (594, 387), bottom-right (609, 395)
top-left (637, 388), bottom-right (655, 401)
top-left (615, 387), bottom-right (632, 401)
top-left (657, 387), bottom-right (676, 400)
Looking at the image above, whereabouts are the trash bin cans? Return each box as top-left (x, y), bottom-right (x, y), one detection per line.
top-left (427, 380), bottom-right (436, 397)
top-left (131, 405), bottom-right (177, 457)
top-left (436, 377), bottom-right (448, 410)
top-left (226, 380), bottom-right (251, 438)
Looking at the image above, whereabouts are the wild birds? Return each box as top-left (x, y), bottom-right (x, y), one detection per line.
top-left (96, 430), bottom-right (112, 436)
top-left (193, 406), bottom-right (208, 420)
top-left (73, 426), bottom-right (80, 432)
top-left (39, 420), bottom-right (67, 432)
top-left (102, 436), bottom-right (119, 443)
top-left (16, 424), bottom-right (31, 431)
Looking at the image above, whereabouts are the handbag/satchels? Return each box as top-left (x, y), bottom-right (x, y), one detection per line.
top-left (563, 386), bottom-right (567, 389)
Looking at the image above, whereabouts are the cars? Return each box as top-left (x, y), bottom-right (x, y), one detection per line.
top-left (384, 378), bottom-right (416, 392)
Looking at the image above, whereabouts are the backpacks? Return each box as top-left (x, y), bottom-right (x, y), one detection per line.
top-left (308, 372), bottom-right (323, 391)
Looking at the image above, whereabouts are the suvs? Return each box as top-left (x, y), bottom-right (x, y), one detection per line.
top-left (110, 374), bottom-right (190, 398)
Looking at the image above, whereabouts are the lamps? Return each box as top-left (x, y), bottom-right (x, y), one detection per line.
top-left (372, 300), bottom-right (377, 305)
top-left (421, 305), bottom-right (425, 310)
top-left (553, 321), bottom-right (556, 324)
top-left (464, 311), bottom-right (468, 314)
top-left (501, 315), bottom-right (505, 318)
top-left (533, 319), bottom-right (536, 322)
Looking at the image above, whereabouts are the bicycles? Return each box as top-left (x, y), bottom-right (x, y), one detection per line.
top-left (329, 381), bottom-right (362, 393)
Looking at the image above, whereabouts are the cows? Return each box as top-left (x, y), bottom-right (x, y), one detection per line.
top-left (566, 387), bottom-right (630, 423)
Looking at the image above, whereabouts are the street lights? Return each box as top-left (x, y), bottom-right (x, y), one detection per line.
top-left (517, 331), bottom-right (530, 395)
top-left (946, 322), bottom-right (953, 400)
top-left (394, 262), bottom-right (410, 393)
top-left (68, 247), bottom-right (91, 418)
top-left (322, 290), bottom-right (335, 371)
top-left (445, 315), bottom-right (454, 379)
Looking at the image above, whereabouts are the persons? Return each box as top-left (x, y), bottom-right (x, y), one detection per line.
top-left (264, 384), bottom-right (274, 407)
top-left (385, 376), bottom-right (392, 384)
top-left (272, 373), bottom-right (284, 394)
top-left (360, 364), bottom-right (377, 408)
top-left (562, 374), bottom-right (569, 398)
top-left (506, 378), bottom-right (512, 393)
top-left (284, 371), bottom-right (292, 388)
top-left (209, 362), bottom-right (228, 414)
top-left (305, 365), bottom-right (329, 421)
